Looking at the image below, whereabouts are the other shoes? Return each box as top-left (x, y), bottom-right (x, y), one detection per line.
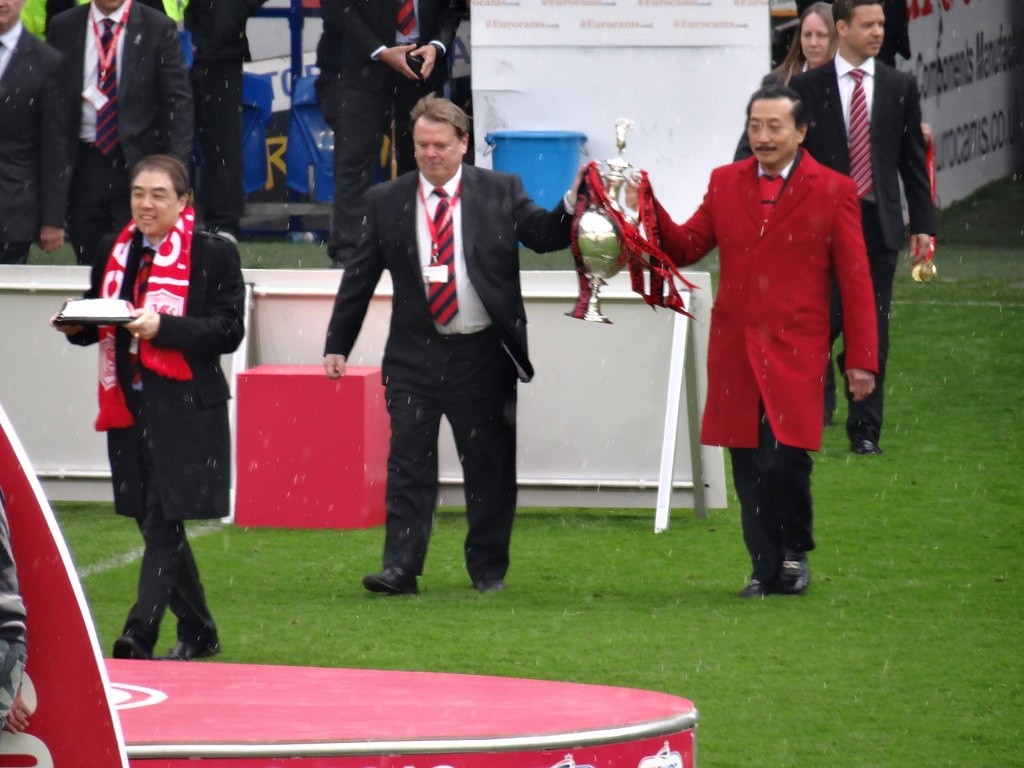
top-left (331, 246), bottom-right (365, 269)
top-left (208, 223), bottom-right (241, 246)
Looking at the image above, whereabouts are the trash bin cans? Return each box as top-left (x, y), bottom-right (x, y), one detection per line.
top-left (483, 131), bottom-right (589, 247)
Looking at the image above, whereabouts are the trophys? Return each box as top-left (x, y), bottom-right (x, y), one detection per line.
top-left (563, 117), bottom-right (643, 324)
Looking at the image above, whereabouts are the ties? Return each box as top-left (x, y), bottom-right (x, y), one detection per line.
top-left (428, 185), bottom-right (458, 326)
top-left (848, 68), bottom-right (873, 197)
top-left (397, 0), bottom-right (416, 36)
top-left (126, 254), bottom-right (147, 390)
top-left (96, 19), bottom-right (118, 155)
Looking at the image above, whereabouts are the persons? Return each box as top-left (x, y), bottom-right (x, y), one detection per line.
top-left (48, 157), bottom-right (245, 660)
top-left (314, 0), bottom-right (465, 269)
top-left (0, 0), bottom-right (194, 264)
top-left (0, 489), bottom-right (32, 735)
top-left (137, 0), bottom-right (269, 246)
top-left (733, 0), bottom-right (932, 162)
top-left (631, 85), bottom-right (879, 599)
top-left (795, 0), bottom-right (911, 69)
top-left (322, 93), bottom-right (588, 595)
top-left (732, 0), bottom-right (935, 454)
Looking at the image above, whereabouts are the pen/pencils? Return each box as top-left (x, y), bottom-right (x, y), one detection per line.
top-left (760, 219), bottom-right (768, 236)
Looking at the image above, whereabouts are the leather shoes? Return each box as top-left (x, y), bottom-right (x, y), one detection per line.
top-left (475, 578), bottom-right (506, 593)
top-left (161, 638), bottom-right (221, 661)
top-left (111, 636), bottom-right (152, 661)
top-left (738, 579), bottom-right (772, 598)
top-left (782, 550), bottom-right (810, 596)
top-left (851, 438), bottom-right (882, 456)
top-left (363, 566), bottom-right (417, 594)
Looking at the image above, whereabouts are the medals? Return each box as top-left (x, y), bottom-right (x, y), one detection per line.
top-left (912, 259), bottom-right (936, 283)
